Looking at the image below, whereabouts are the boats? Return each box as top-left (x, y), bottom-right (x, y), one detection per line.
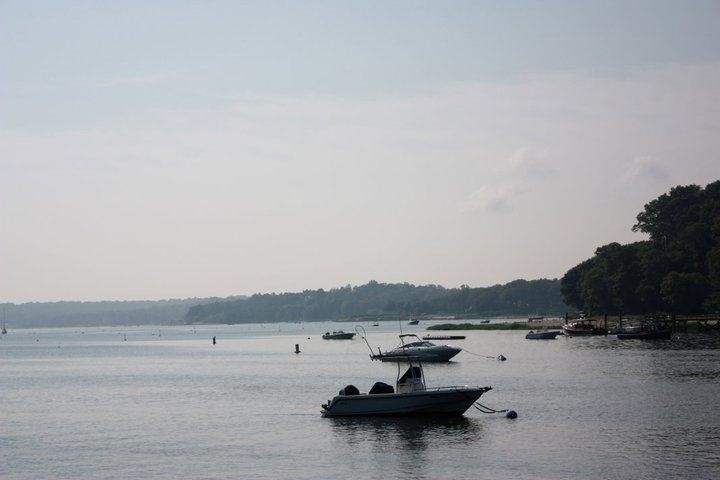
top-left (322, 331), bottom-right (354, 339)
top-left (409, 318), bottom-right (419, 324)
top-left (321, 365), bottom-right (493, 417)
top-left (526, 319), bottom-right (668, 340)
top-left (370, 334), bottom-right (466, 361)
